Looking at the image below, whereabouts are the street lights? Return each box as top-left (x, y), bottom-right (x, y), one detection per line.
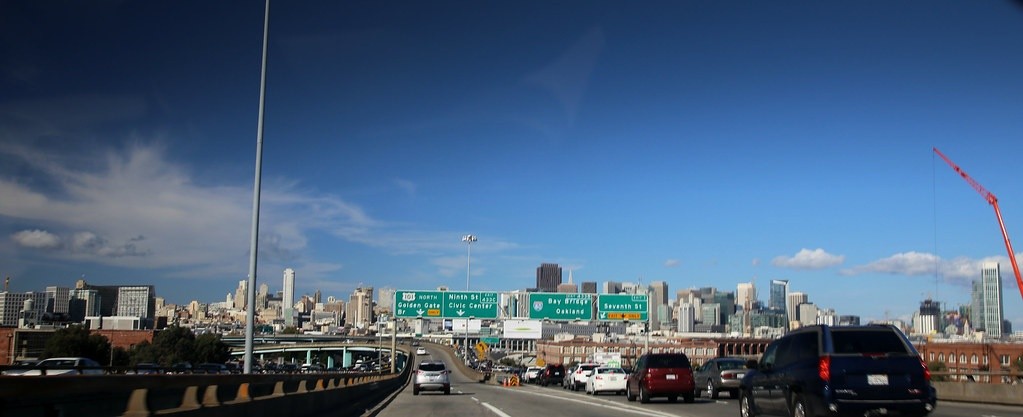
top-left (460, 234), bottom-right (478, 294)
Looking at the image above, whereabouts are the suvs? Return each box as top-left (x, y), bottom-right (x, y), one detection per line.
top-left (738, 321), bottom-right (937, 417)
top-left (410, 360), bottom-right (452, 396)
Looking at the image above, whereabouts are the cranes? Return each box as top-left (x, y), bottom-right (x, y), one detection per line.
top-left (928, 147), bottom-right (1023, 300)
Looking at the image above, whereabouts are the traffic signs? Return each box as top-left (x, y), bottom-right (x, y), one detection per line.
top-left (395, 290), bottom-right (442, 319)
top-left (529, 293), bottom-right (592, 321)
top-left (598, 294), bottom-right (649, 321)
top-left (442, 291), bottom-right (497, 320)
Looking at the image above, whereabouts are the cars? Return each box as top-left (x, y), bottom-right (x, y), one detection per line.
top-left (417, 347), bottom-right (426, 356)
top-left (127, 358), bottom-right (382, 377)
top-left (539, 363), bottom-right (566, 386)
top-left (451, 344), bottom-right (545, 384)
top-left (562, 367), bottom-right (575, 391)
top-left (570, 363), bottom-right (601, 390)
top-left (0, 357), bottom-right (43, 377)
top-left (19, 356), bottom-right (106, 378)
top-left (410, 340), bottom-right (420, 347)
top-left (366, 339), bottom-right (375, 344)
top-left (694, 357), bottom-right (747, 399)
top-left (342, 339), bottom-right (353, 344)
top-left (624, 352), bottom-right (695, 403)
top-left (584, 367), bottom-right (631, 395)
top-left (396, 338), bottom-right (404, 345)
